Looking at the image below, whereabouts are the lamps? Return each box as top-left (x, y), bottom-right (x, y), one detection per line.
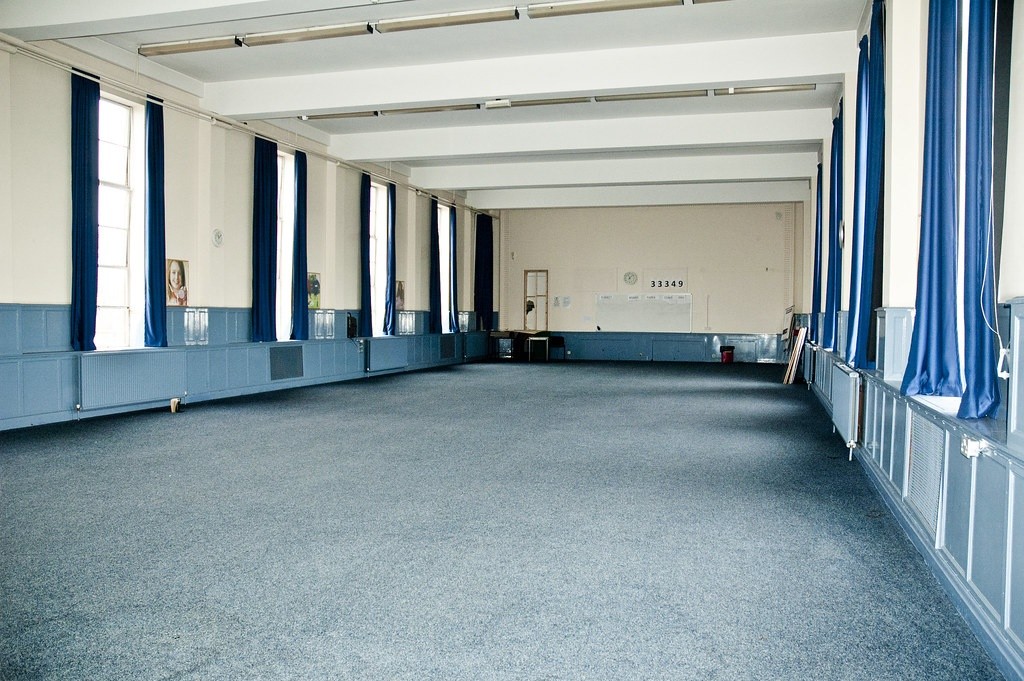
top-left (136, 0), bottom-right (817, 122)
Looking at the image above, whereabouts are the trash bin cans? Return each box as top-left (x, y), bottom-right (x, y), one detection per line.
top-left (720, 346), bottom-right (735, 363)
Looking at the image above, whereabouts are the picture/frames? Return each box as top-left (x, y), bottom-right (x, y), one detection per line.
top-left (396, 281), bottom-right (406, 310)
top-left (166, 259), bottom-right (190, 307)
top-left (307, 272), bottom-right (321, 310)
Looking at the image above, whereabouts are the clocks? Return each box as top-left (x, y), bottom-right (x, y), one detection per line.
top-left (210, 226), bottom-right (226, 248)
top-left (624, 272), bottom-right (637, 285)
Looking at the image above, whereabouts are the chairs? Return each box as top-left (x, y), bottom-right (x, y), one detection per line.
top-left (549, 336), bottom-right (565, 363)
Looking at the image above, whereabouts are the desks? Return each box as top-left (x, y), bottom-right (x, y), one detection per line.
top-left (528, 337), bottom-right (549, 362)
top-left (490, 330), bottom-right (550, 362)
